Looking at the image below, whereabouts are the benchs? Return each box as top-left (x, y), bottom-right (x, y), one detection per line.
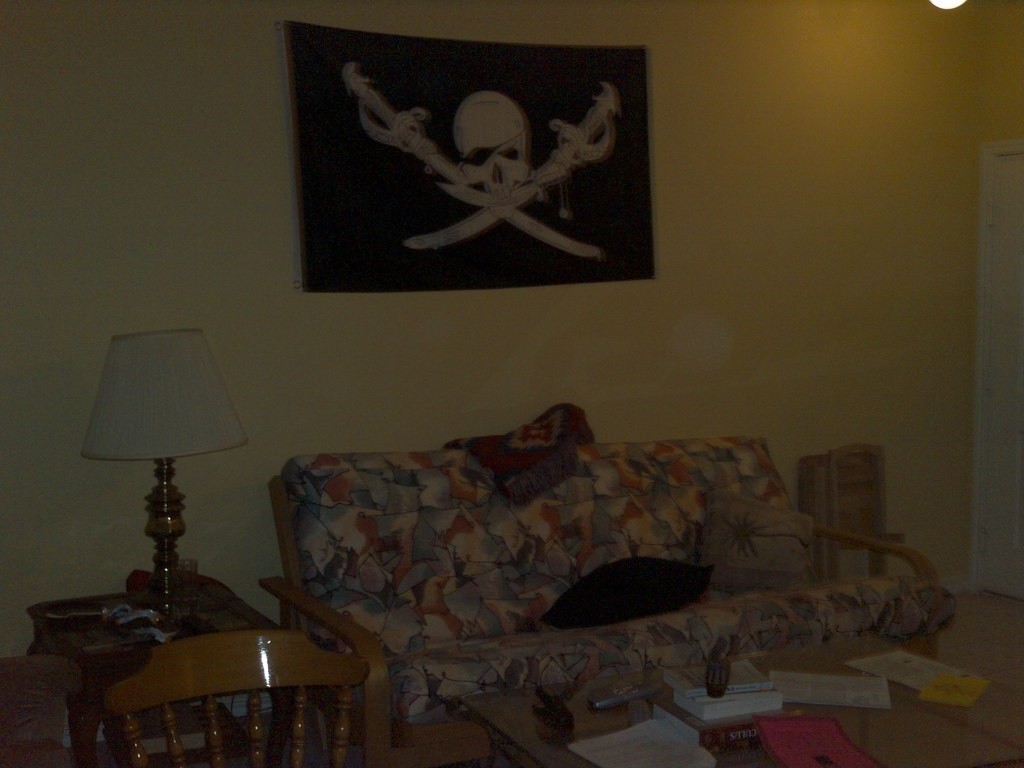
top-left (259, 435), bottom-right (957, 768)
top-left (0, 655), bottom-right (82, 768)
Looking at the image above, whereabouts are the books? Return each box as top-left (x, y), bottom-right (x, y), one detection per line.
top-left (651, 690), bottom-right (785, 750)
top-left (662, 657), bottom-right (773, 696)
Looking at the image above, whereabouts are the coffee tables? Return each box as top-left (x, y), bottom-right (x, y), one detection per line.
top-left (458, 635), bottom-right (1024, 768)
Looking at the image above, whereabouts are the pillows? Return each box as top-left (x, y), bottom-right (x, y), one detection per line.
top-left (540, 557), bottom-right (716, 630)
top-left (703, 490), bottom-right (815, 596)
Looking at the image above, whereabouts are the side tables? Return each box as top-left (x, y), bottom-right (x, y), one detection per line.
top-left (25, 583), bottom-right (285, 768)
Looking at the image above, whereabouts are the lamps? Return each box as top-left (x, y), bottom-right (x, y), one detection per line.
top-left (80, 330), bottom-right (247, 633)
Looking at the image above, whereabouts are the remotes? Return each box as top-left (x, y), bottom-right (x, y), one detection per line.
top-left (704, 658), bottom-right (729, 698)
top-left (587, 684), bottom-right (664, 710)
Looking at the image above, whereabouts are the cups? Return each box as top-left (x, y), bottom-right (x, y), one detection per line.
top-left (176, 559), bottom-right (199, 597)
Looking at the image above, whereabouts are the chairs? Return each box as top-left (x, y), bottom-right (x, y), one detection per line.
top-left (101, 629), bottom-right (371, 768)
